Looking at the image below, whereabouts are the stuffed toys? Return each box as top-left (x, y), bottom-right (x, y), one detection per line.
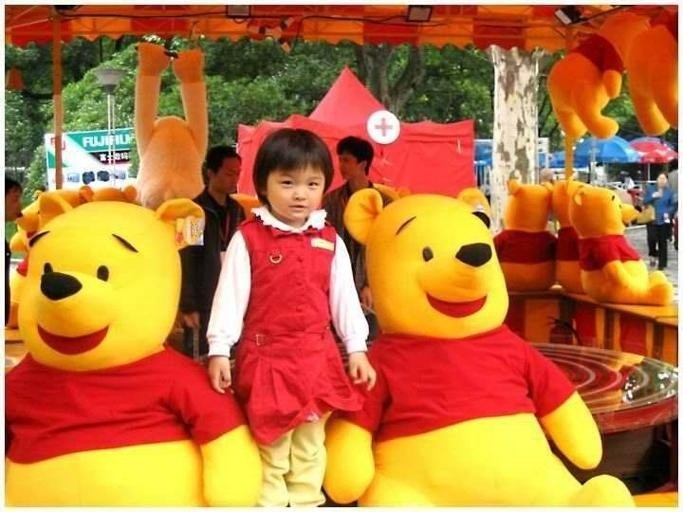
top-left (490, 179), bottom-right (557, 293)
top-left (544, 11), bottom-right (649, 140)
top-left (6, 185), bottom-right (79, 329)
top-left (322, 186), bottom-right (638, 508)
top-left (566, 182), bottom-right (673, 306)
top-left (6, 192), bottom-right (264, 508)
top-left (132, 41), bottom-right (209, 211)
top-left (626, 4), bottom-right (678, 135)
top-left (551, 181), bottom-right (594, 294)
top-left (79, 185), bottom-right (141, 205)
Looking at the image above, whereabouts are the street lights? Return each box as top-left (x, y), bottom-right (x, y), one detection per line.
top-left (93, 66), bottom-right (128, 173)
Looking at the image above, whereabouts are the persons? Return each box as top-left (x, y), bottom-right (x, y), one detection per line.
top-left (5, 176), bottom-right (22, 326)
top-left (321, 136), bottom-right (393, 372)
top-left (641, 171), bottom-right (672, 270)
top-left (206, 126), bottom-right (379, 508)
top-left (175, 145), bottom-right (246, 359)
top-left (668, 167), bottom-right (678, 252)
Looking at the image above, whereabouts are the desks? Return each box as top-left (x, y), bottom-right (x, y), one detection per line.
top-left (503, 282), bottom-right (678, 369)
top-left (525, 341), bottom-right (679, 496)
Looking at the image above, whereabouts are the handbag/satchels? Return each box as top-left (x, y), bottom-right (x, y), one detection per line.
top-left (636, 205), bottom-right (655, 224)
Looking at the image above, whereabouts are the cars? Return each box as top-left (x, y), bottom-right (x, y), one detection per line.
top-left (610, 181), bottom-right (646, 195)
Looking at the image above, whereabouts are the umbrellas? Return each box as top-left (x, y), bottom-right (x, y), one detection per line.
top-left (539, 135), bottom-right (678, 183)
